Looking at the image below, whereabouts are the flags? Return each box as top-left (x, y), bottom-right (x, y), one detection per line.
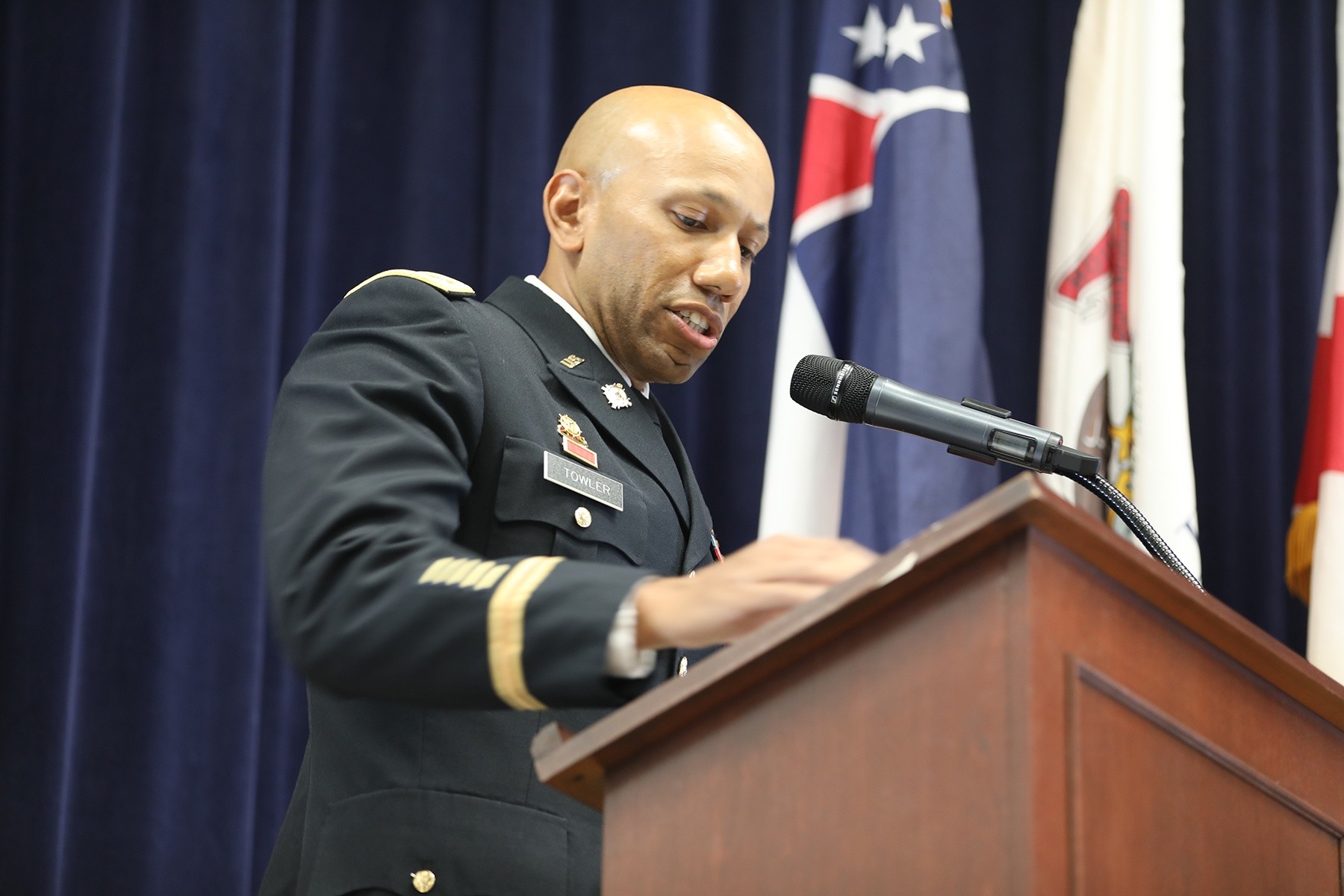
top-left (1033, 1), bottom-right (1209, 590)
top-left (757, 1), bottom-right (1005, 558)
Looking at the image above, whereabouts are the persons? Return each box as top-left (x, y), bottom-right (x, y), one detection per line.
top-left (258, 86), bottom-right (882, 896)
top-left (1284, 174), bottom-right (1344, 684)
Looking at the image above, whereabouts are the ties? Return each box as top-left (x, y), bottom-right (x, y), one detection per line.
top-left (629, 386), bottom-right (664, 441)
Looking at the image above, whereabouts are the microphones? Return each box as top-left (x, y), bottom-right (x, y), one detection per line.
top-left (789, 354), bottom-right (1099, 475)
